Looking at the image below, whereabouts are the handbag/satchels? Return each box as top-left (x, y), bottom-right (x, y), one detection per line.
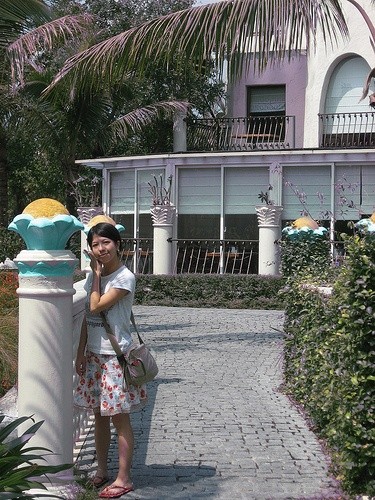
top-left (120, 342), bottom-right (158, 392)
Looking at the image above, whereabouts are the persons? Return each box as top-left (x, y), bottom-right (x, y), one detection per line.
top-left (72, 222), bottom-right (147, 497)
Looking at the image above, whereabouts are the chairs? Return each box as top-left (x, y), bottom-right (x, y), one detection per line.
top-left (233, 256), bottom-right (249, 270)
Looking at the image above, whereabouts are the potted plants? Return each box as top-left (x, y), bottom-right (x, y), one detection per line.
top-left (148, 173), bottom-right (176, 223)
top-left (255, 184), bottom-right (284, 225)
top-left (66, 171), bottom-right (103, 223)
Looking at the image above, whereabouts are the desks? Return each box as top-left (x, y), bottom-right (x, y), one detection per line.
top-left (232, 134), bottom-right (274, 137)
top-left (208, 253), bottom-right (239, 257)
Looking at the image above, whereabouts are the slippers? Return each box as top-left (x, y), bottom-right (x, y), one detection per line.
top-left (92, 473), bottom-right (108, 486)
top-left (99, 486), bottom-right (133, 497)
